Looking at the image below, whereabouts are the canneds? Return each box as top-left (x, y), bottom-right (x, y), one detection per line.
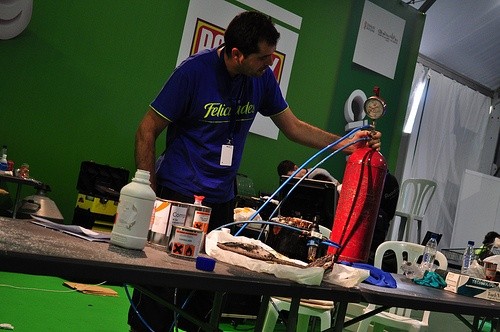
top-left (170, 225), bottom-right (203, 261)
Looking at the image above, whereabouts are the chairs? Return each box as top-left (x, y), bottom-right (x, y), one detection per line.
top-left (356, 240), bottom-right (447, 332)
top-left (261, 218), bottom-right (332, 332)
top-left (385, 178), bottom-right (437, 245)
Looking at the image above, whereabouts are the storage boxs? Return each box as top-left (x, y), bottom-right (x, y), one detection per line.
top-left (72, 161), bottom-right (131, 232)
top-left (264, 175), bottom-right (339, 264)
top-left (444, 270), bottom-right (500, 304)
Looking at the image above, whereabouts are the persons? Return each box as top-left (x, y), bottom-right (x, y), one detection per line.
top-left (475, 231), bottom-right (500, 254)
top-left (128, 10), bottom-right (381, 332)
top-left (278, 160), bottom-right (340, 187)
top-left (367, 167), bottom-right (399, 267)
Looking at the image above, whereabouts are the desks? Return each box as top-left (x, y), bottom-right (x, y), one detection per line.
top-left (0, 173), bottom-right (44, 217)
top-left (0, 218), bottom-right (500, 332)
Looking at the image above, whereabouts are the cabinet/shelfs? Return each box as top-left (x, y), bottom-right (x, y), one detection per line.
top-left (451, 167), bottom-right (499, 255)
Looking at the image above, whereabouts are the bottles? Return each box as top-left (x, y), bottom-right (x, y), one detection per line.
top-left (109, 169), bottom-right (156, 251)
top-left (421, 234), bottom-right (438, 270)
top-left (460, 241), bottom-right (478, 275)
top-left (20, 162), bottom-right (29, 180)
top-left (0, 154), bottom-right (8, 174)
